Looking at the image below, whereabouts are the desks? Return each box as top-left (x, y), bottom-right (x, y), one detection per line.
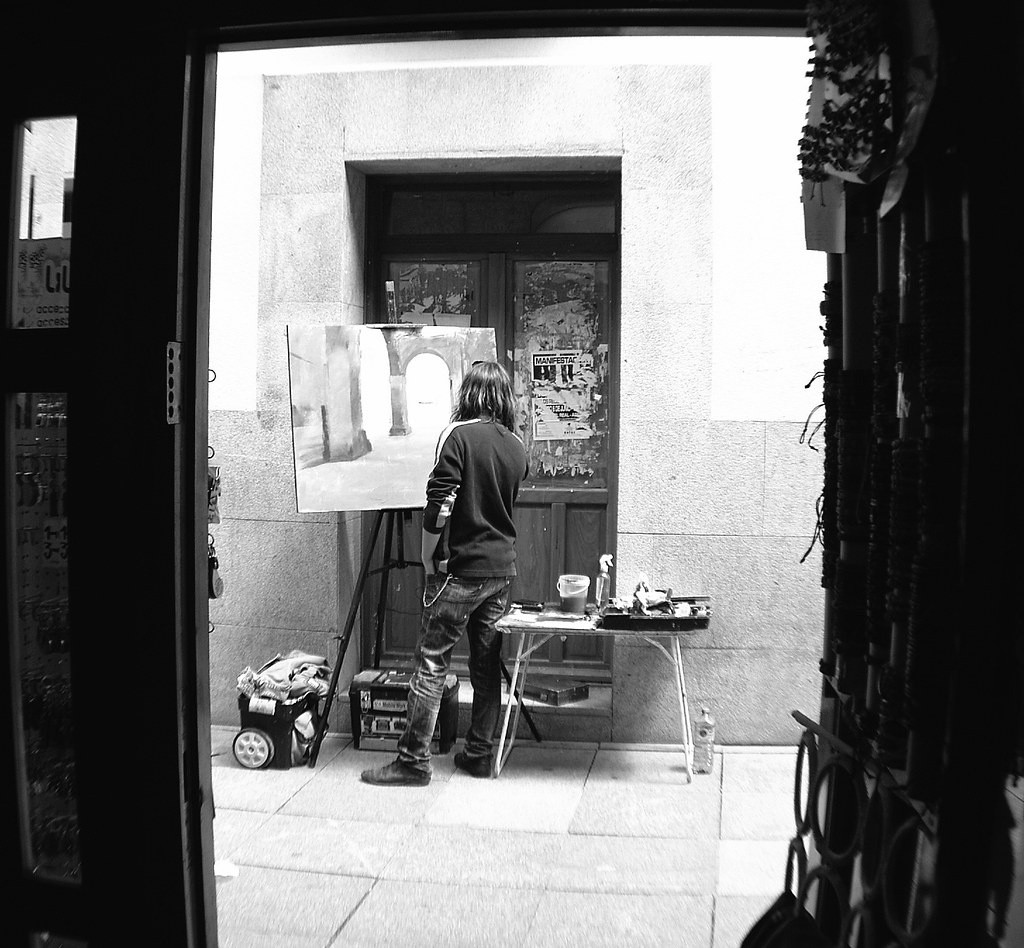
top-left (495, 603), bottom-right (696, 783)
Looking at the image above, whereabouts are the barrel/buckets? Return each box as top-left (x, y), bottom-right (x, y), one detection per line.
top-left (557, 574), bottom-right (591, 613)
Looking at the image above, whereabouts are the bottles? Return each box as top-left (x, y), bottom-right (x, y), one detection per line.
top-left (692, 708), bottom-right (715, 775)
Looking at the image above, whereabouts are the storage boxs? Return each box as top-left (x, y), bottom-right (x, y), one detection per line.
top-left (349, 671), bottom-right (460, 754)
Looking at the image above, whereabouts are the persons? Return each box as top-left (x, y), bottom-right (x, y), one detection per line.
top-left (361, 361), bottom-right (529, 787)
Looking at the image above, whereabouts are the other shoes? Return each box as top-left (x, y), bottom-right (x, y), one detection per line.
top-left (361, 758), bottom-right (431, 786)
top-left (454, 752), bottom-right (491, 778)
